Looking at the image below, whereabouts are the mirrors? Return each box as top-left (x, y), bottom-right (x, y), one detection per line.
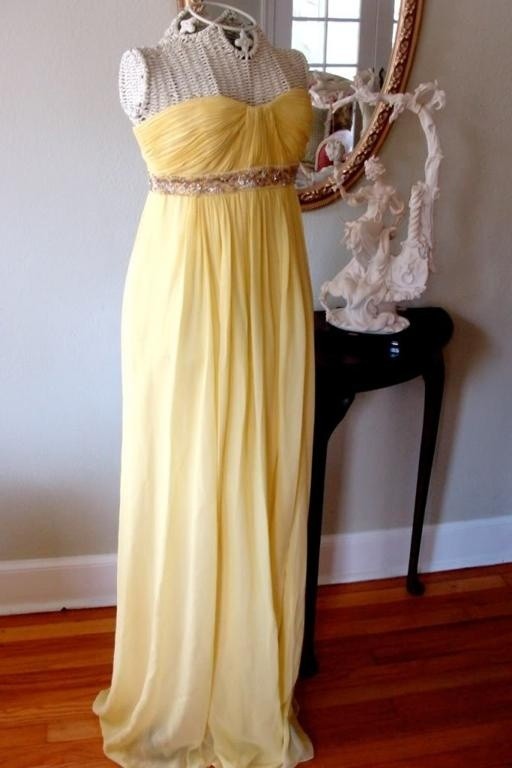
top-left (177, 1), bottom-right (424, 212)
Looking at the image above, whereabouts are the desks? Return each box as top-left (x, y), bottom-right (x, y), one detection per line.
top-left (299, 307), bottom-right (453, 680)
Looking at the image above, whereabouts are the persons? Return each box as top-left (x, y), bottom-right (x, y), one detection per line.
top-left (93, 0), bottom-right (313, 766)
top-left (297, 71), bottom-right (363, 187)
top-left (320, 154), bottom-right (411, 335)
top-left (300, 140), bottom-right (345, 184)
